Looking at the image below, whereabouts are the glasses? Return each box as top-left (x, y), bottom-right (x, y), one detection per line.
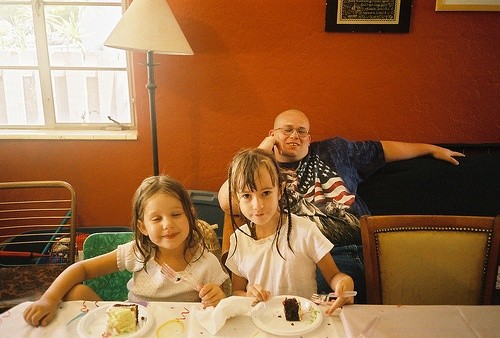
top-left (273, 128), bottom-right (311, 139)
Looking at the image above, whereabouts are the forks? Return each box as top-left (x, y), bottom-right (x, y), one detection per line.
top-left (158, 262), bottom-right (202, 292)
top-left (311, 290), bottom-right (357, 304)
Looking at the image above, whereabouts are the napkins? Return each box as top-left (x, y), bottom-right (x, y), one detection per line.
top-left (193, 296), bottom-right (254, 334)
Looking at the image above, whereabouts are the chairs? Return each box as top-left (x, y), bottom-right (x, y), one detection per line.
top-left (0, 180), bottom-right (77, 293)
top-left (359, 215), bottom-right (500, 305)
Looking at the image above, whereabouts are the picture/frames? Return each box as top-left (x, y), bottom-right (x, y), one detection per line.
top-left (325, 0), bottom-right (412, 32)
top-left (434, 0), bottom-right (500, 12)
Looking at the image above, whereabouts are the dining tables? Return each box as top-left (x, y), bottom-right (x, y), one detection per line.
top-left (0, 302), bottom-right (500, 337)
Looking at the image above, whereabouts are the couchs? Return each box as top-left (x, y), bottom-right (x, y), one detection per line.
top-left (364, 141), bottom-right (500, 214)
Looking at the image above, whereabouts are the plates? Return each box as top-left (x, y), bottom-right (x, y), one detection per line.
top-left (251, 295), bottom-right (323, 336)
top-left (77, 302), bottom-right (154, 338)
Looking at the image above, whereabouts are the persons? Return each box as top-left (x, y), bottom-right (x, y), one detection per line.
top-left (218, 109), bottom-right (465, 305)
top-left (225, 148), bottom-right (355, 315)
top-left (22, 176), bottom-right (232, 327)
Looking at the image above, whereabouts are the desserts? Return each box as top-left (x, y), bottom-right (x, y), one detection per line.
top-left (282, 297), bottom-right (304, 321)
top-left (103, 304), bottom-right (139, 336)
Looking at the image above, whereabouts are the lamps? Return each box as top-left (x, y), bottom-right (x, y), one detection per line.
top-left (103, 0), bottom-right (195, 174)
